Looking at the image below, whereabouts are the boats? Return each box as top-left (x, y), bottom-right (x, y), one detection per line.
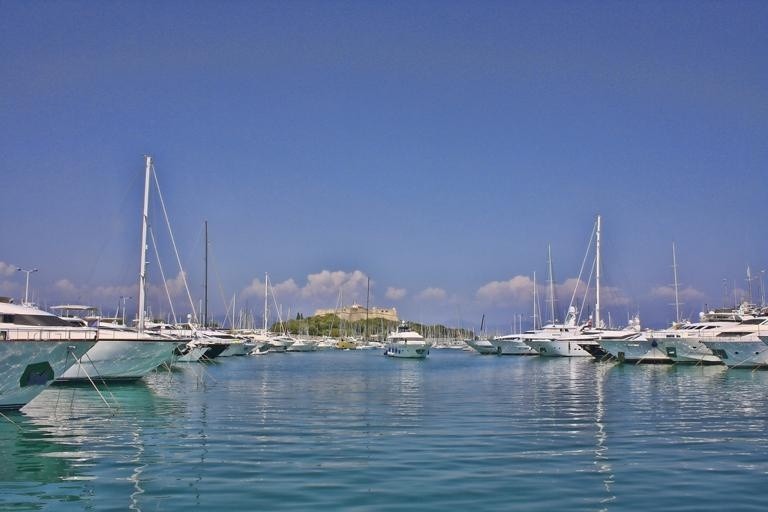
top-left (387, 320), bottom-right (431, 358)
